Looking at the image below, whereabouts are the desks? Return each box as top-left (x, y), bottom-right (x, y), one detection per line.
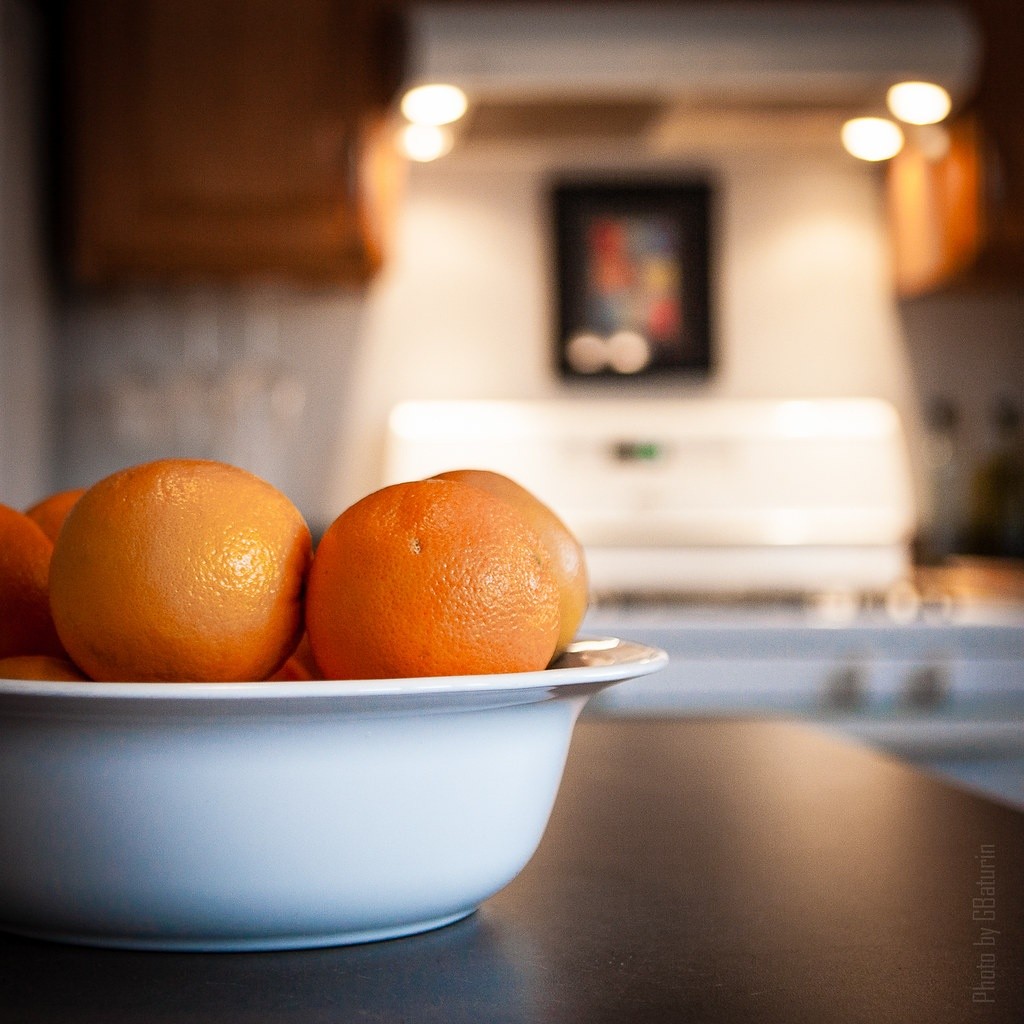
top-left (0, 695), bottom-right (1024, 1024)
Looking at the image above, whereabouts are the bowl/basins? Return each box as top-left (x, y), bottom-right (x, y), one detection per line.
top-left (0, 630), bottom-right (669, 954)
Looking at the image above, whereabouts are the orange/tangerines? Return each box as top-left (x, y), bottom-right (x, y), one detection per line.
top-left (0, 458), bottom-right (591, 681)
